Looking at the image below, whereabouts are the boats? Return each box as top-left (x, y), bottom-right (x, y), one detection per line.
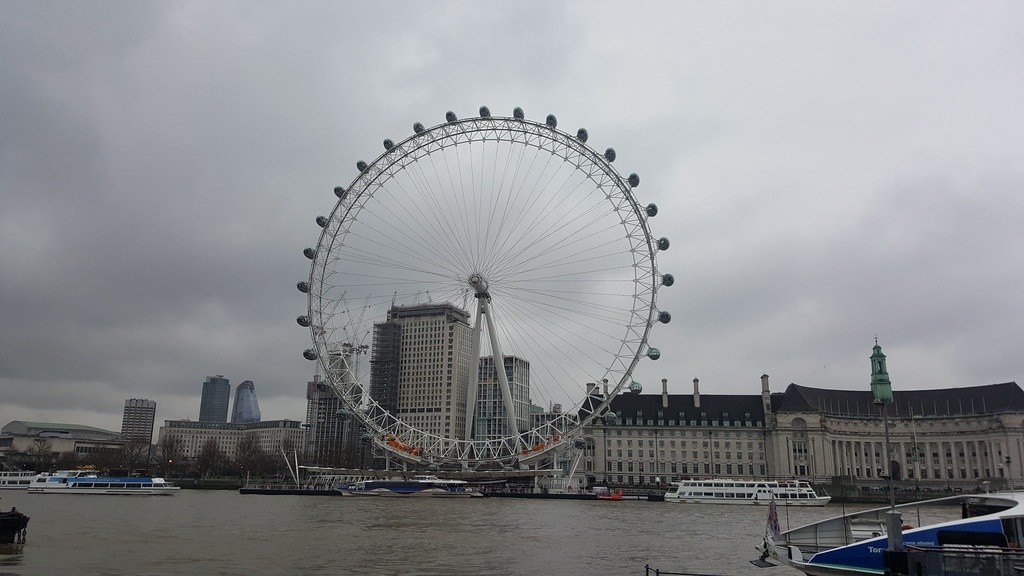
top-left (0, 470), bottom-right (35, 488)
top-left (338, 479), bottom-right (485, 498)
top-left (662, 477), bottom-right (834, 505)
top-left (27, 476), bottom-right (182, 495)
